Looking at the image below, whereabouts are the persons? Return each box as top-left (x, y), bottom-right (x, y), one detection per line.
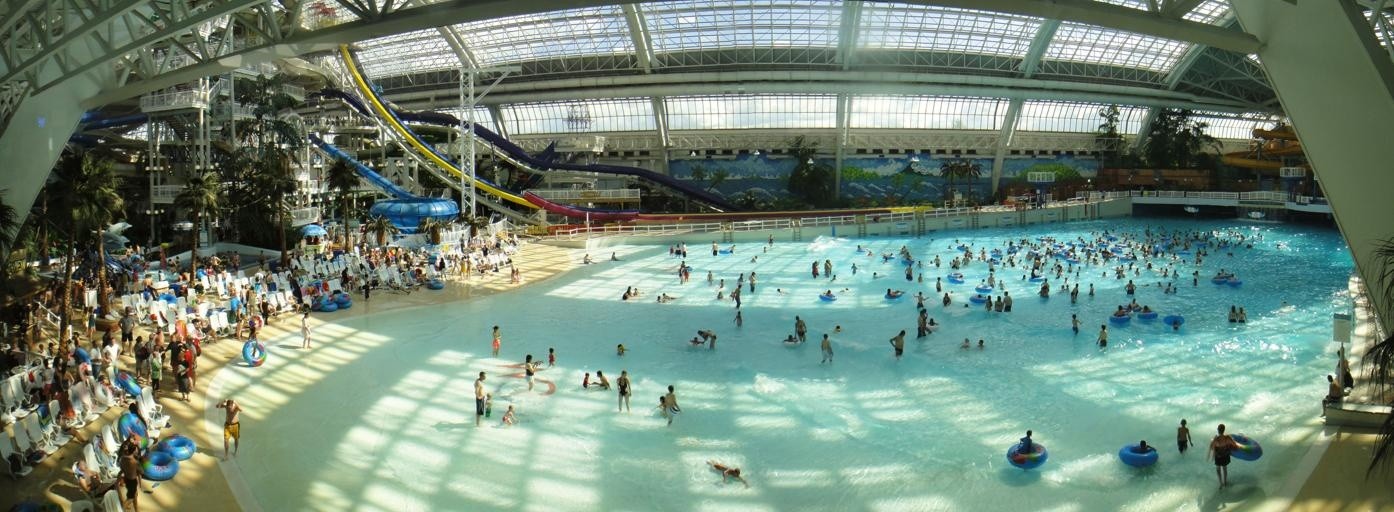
top-left (1176, 417), bottom-right (1195, 456)
top-left (1133, 440), bottom-right (1157, 454)
top-left (1335, 349), bottom-right (1349, 387)
top-left (1205, 423), bottom-right (1238, 492)
top-left (1318, 374), bottom-right (1342, 418)
top-left (1343, 367), bottom-right (1354, 389)
top-left (1018, 428), bottom-right (1034, 473)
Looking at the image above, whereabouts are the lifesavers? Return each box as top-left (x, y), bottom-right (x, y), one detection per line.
top-left (819, 294), bottom-right (837, 301)
top-left (251, 315), bottom-right (262, 329)
top-left (1229, 433), bottom-right (1262, 460)
top-left (139, 451), bottom-right (179, 480)
top-left (719, 250), bottom-right (729, 254)
top-left (1119, 443), bottom-right (1159, 467)
top-left (118, 372), bottom-right (142, 396)
top-left (1164, 315), bottom-right (1184, 325)
top-left (1214, 277), bottom-right (1241, 284)
top-left (311, 292), bottom-right (352, 312)
top-left (971, 284), bottom-right (990, 304)
top-left (119, 414), bottom-right (149, 449)
top-left (1007, 443), bottom-right (1048, 469)
top-left (1138, 311), bottom-right (1157, 318)
top-left (158, 435), bottom-right (196, 461)
top-left (990, 245), bottom-right (1018, 265)
top-left (428, 282), bottom-right (443, 289)
top-left (243, 340), bottom-right (267, 367)
top-left (74, 349), bottom-right (91, 364)
top-left (948, 272), bottom-right (964, 283)
top-left (1054, 235), bottom-right (1135, 262)
top-left (1154, 245), bottom-right (1158, 252)
top-left (1029, 274), bottom-right (1045, 281)
top-left (158, 294), bottom-right (176, 303)
top-left (1109, 315), bottom-right (1129, 322)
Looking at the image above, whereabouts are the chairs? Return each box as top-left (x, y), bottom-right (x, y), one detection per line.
top-left (0, 262), bottom-right (172, 512)
top-left (171, 238), bottom-right (519, 347)
